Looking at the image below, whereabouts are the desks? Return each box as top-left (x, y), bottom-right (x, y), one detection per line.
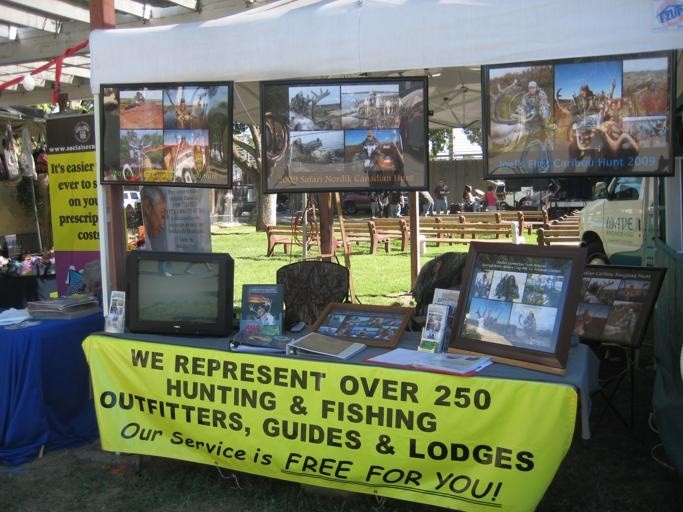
top-left (0, 255), bottom-right (104, 465)
top-left (81, 318), bottom-right (579, 511)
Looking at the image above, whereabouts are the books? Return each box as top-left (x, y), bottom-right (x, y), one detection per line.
top-left (26, 294), bottom-right (102, 319)
top-left (363, 288), bottom-right (494, 376)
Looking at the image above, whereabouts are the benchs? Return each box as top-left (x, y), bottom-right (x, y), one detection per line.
top-left (265, 209), bottom-right (584, 255)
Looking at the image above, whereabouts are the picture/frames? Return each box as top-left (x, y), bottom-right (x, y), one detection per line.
top-left (445, 240), bottom-right (586, 376)
top-left (310, 302), bottom-right (413, 349)
top-left (577, 263), bottom-right (669, 349)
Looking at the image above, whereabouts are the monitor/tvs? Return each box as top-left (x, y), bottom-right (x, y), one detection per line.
top-left (98, 79), bottom-right (233, 189)
top-left (259, 75), bottom-right (429, 192)
top-left (125, 250), bottom-right (234, 338)
top-left (481, 49), bottom-right (677, 179)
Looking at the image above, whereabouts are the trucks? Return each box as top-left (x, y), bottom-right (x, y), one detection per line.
top-left (573, 176), bottom-right (655, 267)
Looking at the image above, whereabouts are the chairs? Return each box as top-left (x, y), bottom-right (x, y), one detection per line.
top-left (276, 260), bottom-right (349, 324)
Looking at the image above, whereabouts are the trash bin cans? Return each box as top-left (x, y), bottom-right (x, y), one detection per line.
top-left (449, 203), bottom-right (461, 214)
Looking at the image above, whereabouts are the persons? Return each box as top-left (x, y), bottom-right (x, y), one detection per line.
top-left (368, 179), bottom-right (500, 217)
top-left (517, 80), bottom-right (639, 173)
top-left (124, 92), bottom-right (192, 172)
top-left (473, 273), bottom-right (643, 341)
top-left (288, 90), bottom-right (410, 187)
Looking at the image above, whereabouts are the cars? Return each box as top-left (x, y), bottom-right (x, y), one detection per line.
top-left (485, 180), bottom-right (533, 210)
top-left (344, 191), bottom-right (408, 217)
top-left (124, 191), bottom-right (141, 207)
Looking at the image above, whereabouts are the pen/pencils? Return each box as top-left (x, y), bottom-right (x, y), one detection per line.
top-left (7, 240), bottom-right (22, 248)
